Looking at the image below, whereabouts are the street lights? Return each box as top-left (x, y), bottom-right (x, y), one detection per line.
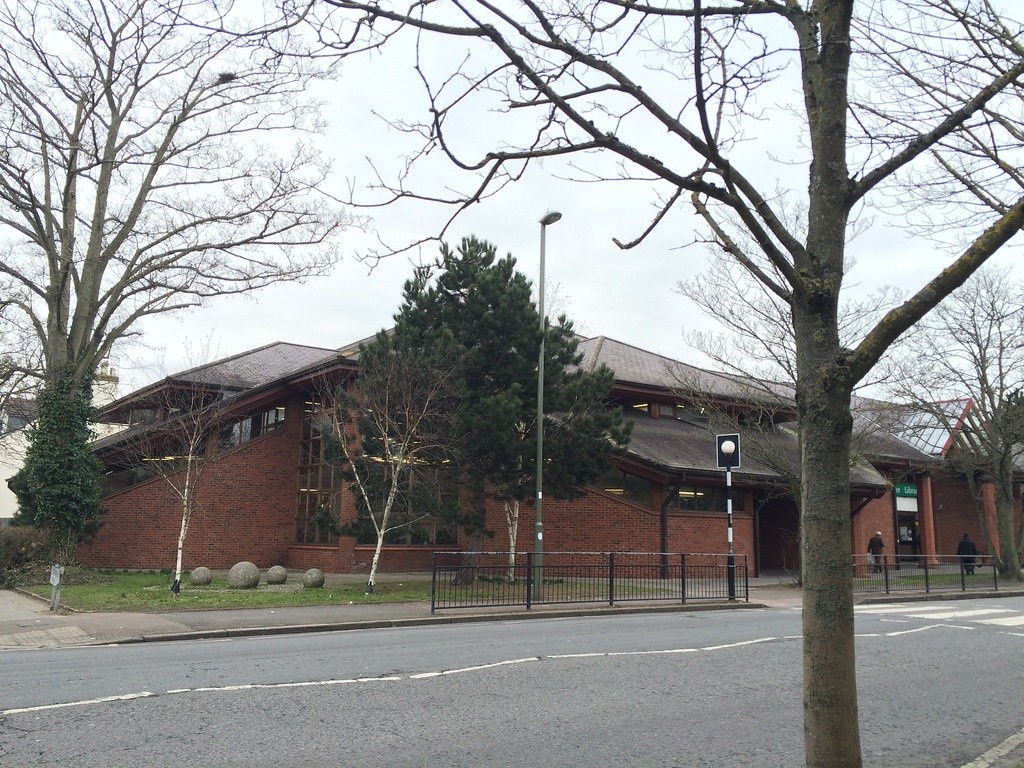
top-left (531, 211), bottom-right (562, 600)
top-left (721, 438), bottom-right (737, 600)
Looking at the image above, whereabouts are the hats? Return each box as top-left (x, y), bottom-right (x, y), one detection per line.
top-left (876, 531), bottom-right (882, 535)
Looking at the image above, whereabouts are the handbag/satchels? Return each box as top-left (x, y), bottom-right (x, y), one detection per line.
top-left (866, 554), bottom-right (875, 572)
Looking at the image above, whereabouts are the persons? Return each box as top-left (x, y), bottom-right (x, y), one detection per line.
top-left (957, 533), bottom-right (977, 575)
top-left (867, 530), bottom-right (886, 573)
